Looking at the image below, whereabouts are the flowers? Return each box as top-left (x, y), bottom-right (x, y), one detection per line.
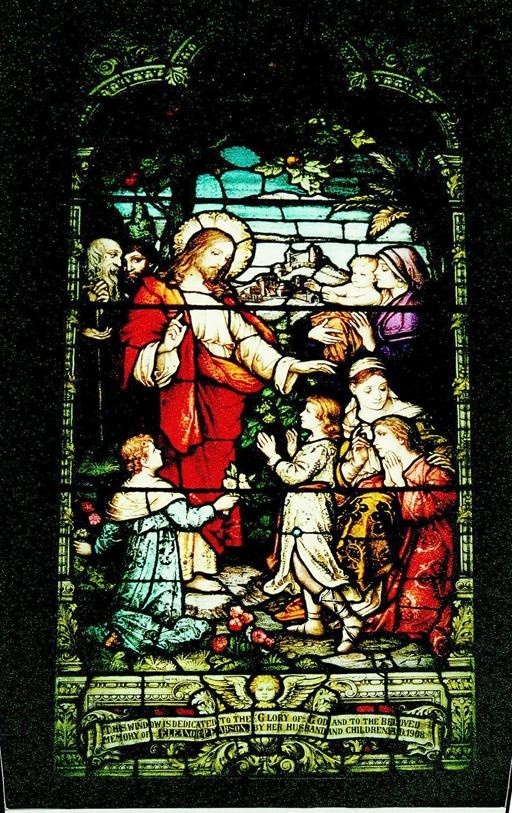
top-left (222, 463), bottom-right (256, 515)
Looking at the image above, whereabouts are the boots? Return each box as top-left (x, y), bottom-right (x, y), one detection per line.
top-left (287, 587), bottom-right (324, 636)
top-left (317, 589), bottom-right (363, 653)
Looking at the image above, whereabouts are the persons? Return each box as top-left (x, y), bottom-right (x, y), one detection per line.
top-left (362, 413), bottom-right (456, 660)
top-left (254, 394), bottom-right (367, 655)
top-left (120, 209), bottom-right (336, 598)
top-left (326, 353), bottom-right (452, 632)
top-left (68, 429), bottom-right (241, 652)
